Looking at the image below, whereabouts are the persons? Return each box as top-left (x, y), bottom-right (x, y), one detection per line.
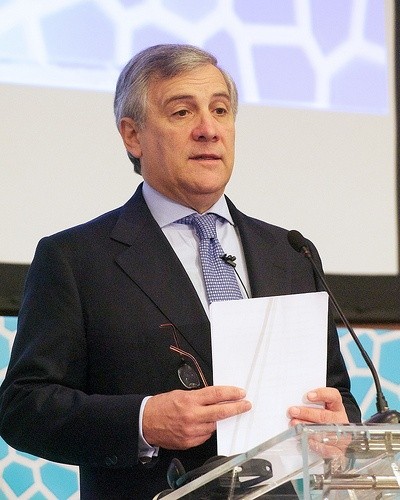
top-left (0, 42), bottom-right (363, 500)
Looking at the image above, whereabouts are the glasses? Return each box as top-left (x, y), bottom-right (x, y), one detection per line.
top-left (159, 323), bottom-right (209, 390)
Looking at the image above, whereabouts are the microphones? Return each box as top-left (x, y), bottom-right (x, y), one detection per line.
top-left (287, 226), bottom-right (400, 424)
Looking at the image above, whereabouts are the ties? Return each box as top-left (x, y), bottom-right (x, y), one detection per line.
top-left (175, 213), bottom-right (244, 303)
